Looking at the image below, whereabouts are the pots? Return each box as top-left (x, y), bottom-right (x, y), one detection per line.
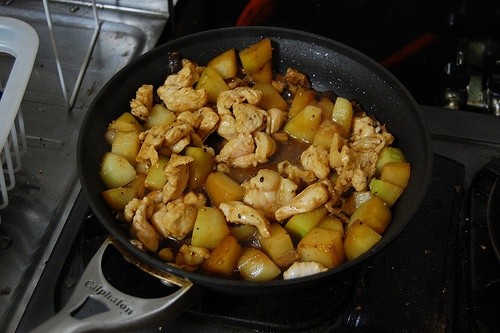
top-left (27, 26), bottom-right (433, 333)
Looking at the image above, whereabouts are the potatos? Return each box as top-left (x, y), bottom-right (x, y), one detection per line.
top-left (98, 38), bottom-right (410, 282)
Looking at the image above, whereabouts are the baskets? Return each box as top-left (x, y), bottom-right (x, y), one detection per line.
top-left (1, 15), bottom-right (40, 211)
